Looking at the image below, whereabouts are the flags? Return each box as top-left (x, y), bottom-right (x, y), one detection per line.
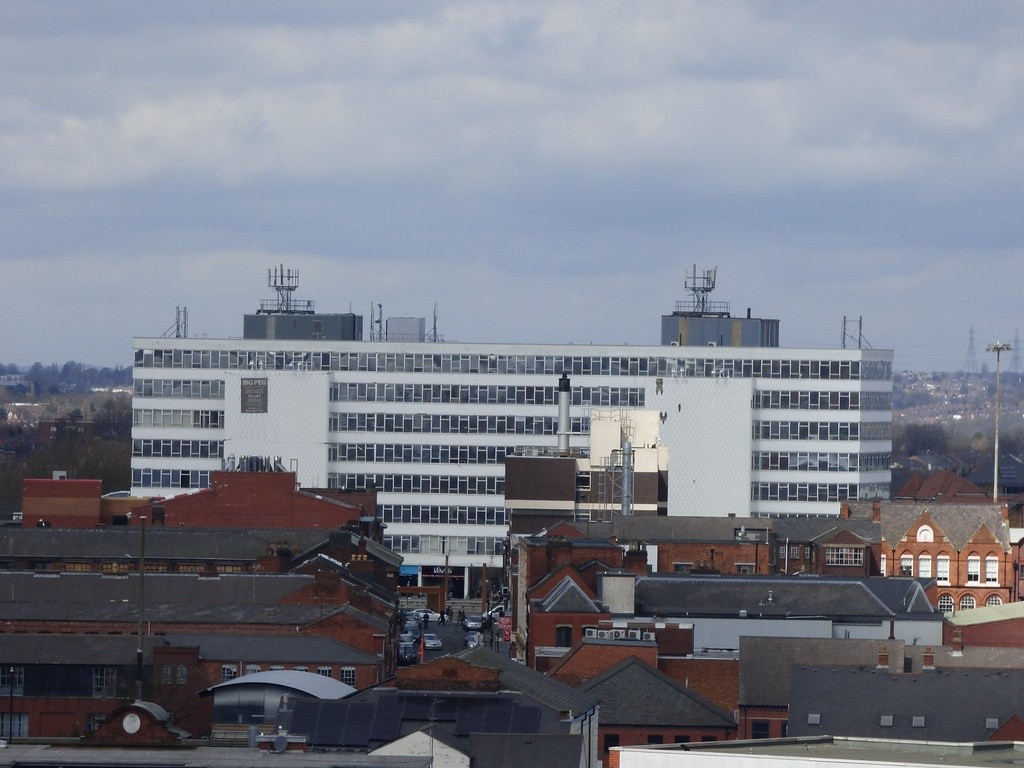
top-left (419, 639), bottom-right (423, 657)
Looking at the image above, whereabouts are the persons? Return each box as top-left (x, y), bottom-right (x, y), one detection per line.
top-left (437, 606), bottom-right (466, 626)
top-left (423, 612), bottom-right (429, 629)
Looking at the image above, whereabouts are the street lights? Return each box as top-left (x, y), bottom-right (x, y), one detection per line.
top-left (985, 341), bottom-right (1013, 503)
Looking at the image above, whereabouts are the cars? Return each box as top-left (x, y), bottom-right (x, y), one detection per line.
top-left (399, 603), bottom-right (507, 665)
